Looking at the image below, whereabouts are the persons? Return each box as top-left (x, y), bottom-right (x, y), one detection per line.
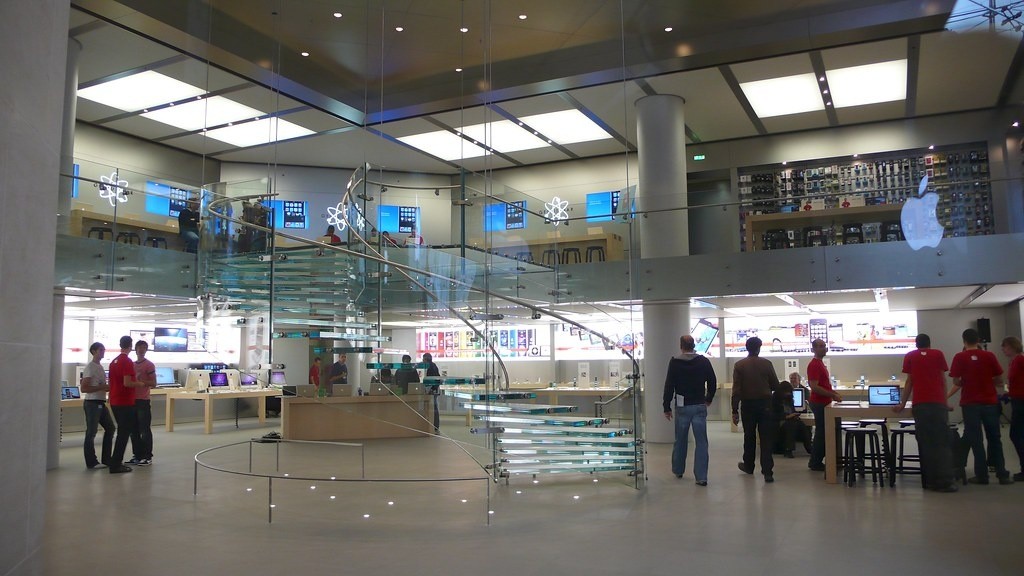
top-left (403, 226), bottom-right (423, 244)
top-left (371, 355), bottom-right (420, 395)
top-left (946, 328), bottom-right (1024, 484)
top-left (325, 225), bottom-right (341, 243)
top-left (731, 337), bottom-right (780, 483)
top-left (662, 334), bottom-right (717, 485)
top-left (892, 332), bottom-right (958, 493)
top-left (771, 372), bottom-right (812, 458)
top-left (842, 198), bottom-right (851, 207)
top-left (179, 198), bottom-right (200, 254)
top-left (804, 202), bottom-right (812, 210)
top-left (808, 339), bottom-right (842, 470)
top-left (423, 353), bottom-right (440, 435)
top-left (109, 335), bottom-right (157, 474)
top-left (238, 198), bottom-right (269, 250)
top-left (309, 353), bottom-right (347, 395)
top-left (371, 227), bottom-right (396, 244)
top-left (80, 341), bottom-right (115, 469)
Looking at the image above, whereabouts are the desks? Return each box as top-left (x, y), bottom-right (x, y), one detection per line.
top-left (69, 209), bottom-right (180, 237)
top-left (746, 203), bottom-right (905, 253)
top-left (480, 233), bottom-right (624, 263)
top-left (165, 389), bottom-right (282, 434)
top-left (439, 383), bottom-right (645, 426)
top-left (823, 400), bottom-right (913, 485)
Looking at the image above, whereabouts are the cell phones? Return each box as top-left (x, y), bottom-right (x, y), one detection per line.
top-left (810, 319), bottom-right (844, 351)
top-left (690, 320), bottom-right (719, 354)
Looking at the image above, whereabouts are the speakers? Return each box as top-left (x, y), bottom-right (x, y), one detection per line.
top-left (976, 318), bottom-right (991, 343)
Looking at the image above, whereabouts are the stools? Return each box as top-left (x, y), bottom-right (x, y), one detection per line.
top-left (765, 220), bottom-right (907, 250)
top-left (515, 250), bottom-right (532, 262)
top-left (87, 227), bottom-right (189, 252)
top-left (840, 419), bottom-right (968, 488)
top-left (586, 246), bottom-right (605, 263)
top-left (562, 247), bottom-right (582, 264)
top-left (541, 248), bottom-right (561, 265)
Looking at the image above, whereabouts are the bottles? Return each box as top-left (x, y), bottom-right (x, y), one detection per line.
top-left (358, 388), bottom-right (362, 396)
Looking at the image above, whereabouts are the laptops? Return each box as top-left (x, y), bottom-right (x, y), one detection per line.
top-left (869, 387), bottom-right (900, 405)
top-left (333, 385), bottom-right (352, 397)
top-left (838, 386), bottom-right (864, 406)
top-left (295, 383), bottom-right (314, 398)
top-left (408, 383), bottom-right (426, 395)
top-left (369, 383), bottom-right (390, 396)
top-left (155, 367), bottom-right (180, 386)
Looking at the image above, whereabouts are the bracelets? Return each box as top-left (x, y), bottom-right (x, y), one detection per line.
top-left (832, 394), bottom-right (834, 397)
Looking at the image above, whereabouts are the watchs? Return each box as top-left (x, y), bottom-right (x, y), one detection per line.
top-left (900, 401), bottom-right (906, 404)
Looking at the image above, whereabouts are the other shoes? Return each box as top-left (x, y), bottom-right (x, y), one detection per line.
top-left (764, 475), bottom-right (775, 482)
top-left (968, 476), bottom-right (989, 484)
top-left (675, 473), bottom-right (682, 479)
top-left (812, 463), bottom-right (826, 470)
top-left (987, 466), bottom-right (996, 472)
top-left (999, 477), bottom-right (1014, 485)
top-left (784, 451), bottom-right (794, 458)
top-left (694, 480), bottom-right (707, 486)
top-left (137, 459), bottom-right (152, 465)
top-left (94, 463), bottom-right (106, 468)
top-left (738, 461), bottom-right (752, 475)
top-left (1013, 472), bottom-right (1024, 482)
top-left (124, 458), bottom-right (140, 465)
top-left (122, 464), bottom-right (132, 472)
top-left (947, 483), bottom-right (960, 492)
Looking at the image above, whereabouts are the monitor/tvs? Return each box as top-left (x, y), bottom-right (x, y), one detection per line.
top-left (61, 387), bottom-right (80, 400)
top-left (130, 327), bottom-right (209, 353)
top-left (792, 388), bottom-right (805, 412)
top-left (184, 368), bottom-right (288, 392)
